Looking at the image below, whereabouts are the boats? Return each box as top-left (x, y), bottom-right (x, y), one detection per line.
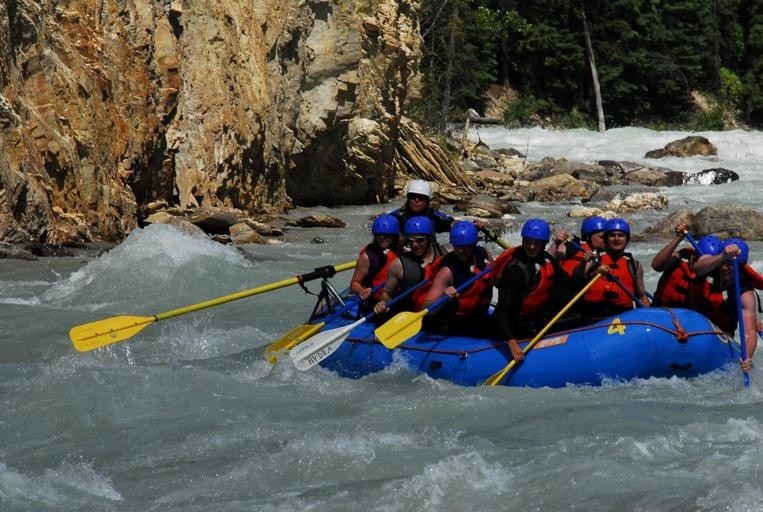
top-left (292, 280), bottom-right (741, 388)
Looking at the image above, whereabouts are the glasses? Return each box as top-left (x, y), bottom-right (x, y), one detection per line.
top-left (407, 237), bottom-right (427, 244)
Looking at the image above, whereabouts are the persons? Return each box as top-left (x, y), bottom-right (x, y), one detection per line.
top-left (348, 180), bottom-right (650, 363)
top-left (651, 224), bottom-right (763, 373)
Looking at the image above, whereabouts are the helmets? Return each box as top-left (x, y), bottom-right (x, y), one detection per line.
top-left (581, 216), bottom-right (607, 235)
top-left (720, 238), bottom-right (749, 264)
top-left (372, 215), bottom-right (400, 235)
top-left (521, 219), bottom-right (550, 243)
top-left (407, 179), bottom-right (433, 200)
top-left (696, 235), bottom-right (724, 256)
top-left (604, 218), bottom-right (630, 234)
top-left (449, 221), bottom-right (478, 247)
top-left (403, 216), bottom-right (433, 235)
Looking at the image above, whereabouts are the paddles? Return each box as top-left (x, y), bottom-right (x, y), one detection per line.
top-left (290, 279), bottom-right (427, 371)
top-left (373, 265), bottom-right (495, 350)
top-left (263, 281), bottom-right (384, 363)
top-left (482, 268), bottom-right (603, 384)
top-left (69, 261), bottom-right (357, 353)
